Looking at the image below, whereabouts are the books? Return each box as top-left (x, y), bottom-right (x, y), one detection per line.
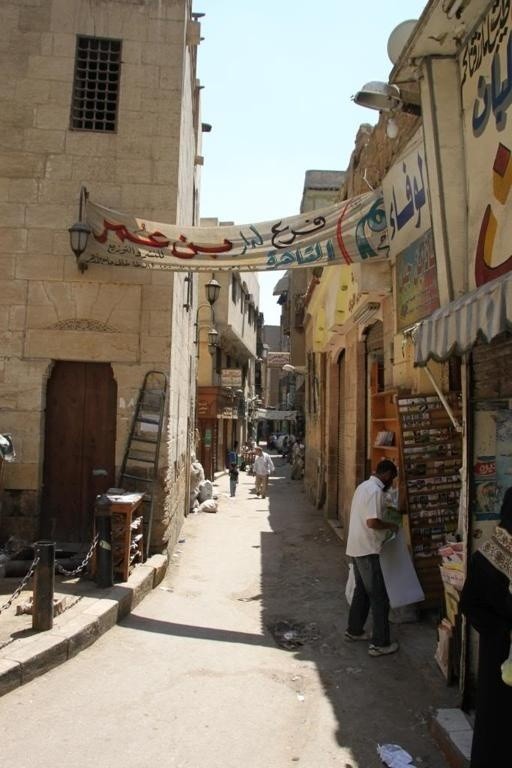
top-left (434, 539), bottom-right (468, 665)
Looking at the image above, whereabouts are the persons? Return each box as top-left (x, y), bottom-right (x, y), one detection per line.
top-left (228, 462), bottom-right (239, 497)
top-left (251, 446), bottom-right (275, 499)
top-left (342, 459), bottom-right (405, 656)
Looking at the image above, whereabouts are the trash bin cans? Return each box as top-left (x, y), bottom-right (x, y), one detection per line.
top-left (226, 452), bottom-right (236, 468)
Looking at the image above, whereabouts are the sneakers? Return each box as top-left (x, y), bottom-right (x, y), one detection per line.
top-left (368, 641), bottom-right (399, 657)
top-left (344, 630), bottom-right (373, 642)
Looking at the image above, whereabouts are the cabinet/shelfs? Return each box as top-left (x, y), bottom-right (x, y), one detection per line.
top-left (369, 390), bottom-right (406, 513)
top-left (90, 490), bottom-right (147, 582)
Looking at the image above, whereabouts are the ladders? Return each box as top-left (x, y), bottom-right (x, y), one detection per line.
top-left (116, 371), bottom-right (168, 563)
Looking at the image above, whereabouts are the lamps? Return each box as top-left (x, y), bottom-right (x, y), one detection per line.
top-left (69, 185), bottom-right (91, 274)
top-left (184, 272), bottom-right (222, 361)
